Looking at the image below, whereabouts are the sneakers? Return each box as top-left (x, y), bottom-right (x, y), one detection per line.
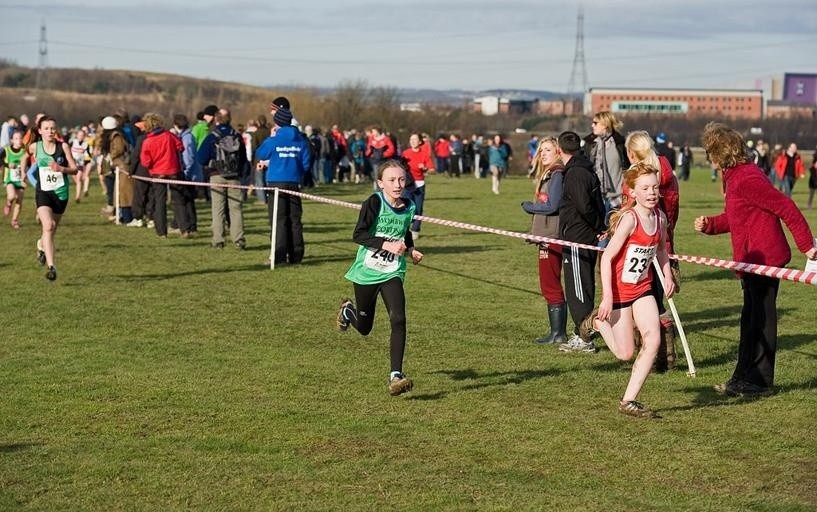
top-left (234, 237), bottom-right (247, 250)
top-left (11, 219), bottom-right (21, 230)
top-left (410, 229), bottom-right (420, 240)
top-left (75, 196), bottom-right (81, 204)
top-left (725, 375), bottom-right (777, 398)
top-left (146, 220), bottom-right (200, 240)
top-left (4, 201), bottom-right (13, 216)
top-left (262, 256), bottom-right (288, 266)
top-left (336, 297), bottom-right (354, 331)
top-left (388, 372), bottom-right (414, 397)
top-left (101, 205), bottom-right (123, 225)
top-left (579, 306), bottom-right (608, 344)
top-left (711, 371), bottom-right (764, 394)
top-left (126, 217), bottom-right (143, 228)
top-left (559, 334), bottom-right (597, 355)
top-left (46, 264), bottom-right (57, 281)
top-left (209, 239), bottom-right (225, 249)
top-left (36, 239), bottom-right (47, 266)
top-left (84, 191), bottom-right (89, 198)
top-left (618, 398), bottom-right (657, 419)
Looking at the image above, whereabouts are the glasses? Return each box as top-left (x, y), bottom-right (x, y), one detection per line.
top-left (592, 122), bottom-right (599, 126)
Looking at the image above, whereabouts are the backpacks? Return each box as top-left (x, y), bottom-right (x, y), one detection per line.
top-left (211, 128), bottom-right (244, 179)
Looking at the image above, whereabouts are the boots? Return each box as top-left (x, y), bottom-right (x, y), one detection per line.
top-left (534, 300), bottom-right (569, 346)
top-left (655, 308), bottom-right (679, 376)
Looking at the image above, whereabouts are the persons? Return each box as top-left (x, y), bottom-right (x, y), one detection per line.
top-left (337, 159), bottom-right (425, 396)
top-left (557, 130), bottom-right (609, 354)
top-left (655, 133), bottom-right (817, 209)
top-left (583, 111), bottom-right (631, 273)
top-left (20, 117), bottom-right (78, 281)
top-left (619, 130), bottom-right (680, 373)
top-left (257, 107), bottom-right (312, 271)
top-left (695, 120), bottom-right (817, 400)
top-left (524, 136), bottom-right (568, 344)
top-left (581, 164), bottom-right (678, 417)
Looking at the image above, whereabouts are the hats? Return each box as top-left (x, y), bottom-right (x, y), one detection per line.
top-left (101, 116), bottom-right (117, 130)
top-left (272, 96), bottom-right (290, 110)
top-left (204, 104), bottom-right (220, 118)
top-left (273, 108), bottom-right (293, 128)
